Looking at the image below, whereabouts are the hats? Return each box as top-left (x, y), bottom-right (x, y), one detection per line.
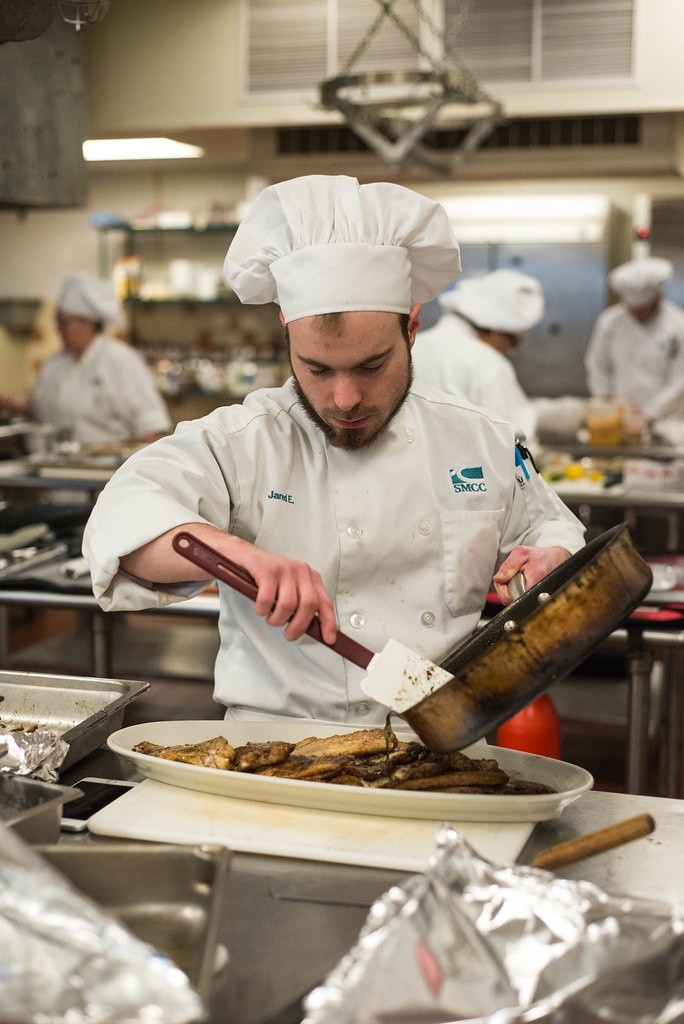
top-left (608, 256), bottom-right (673, 304)
top-left (56, 259), bottom-right (120, 323)
top-left (436, 268), bottom-right (546, 333)
top-left (220, 173), bottom-right (462, 322)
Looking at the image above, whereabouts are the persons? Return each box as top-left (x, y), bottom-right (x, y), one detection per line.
top-left (411, 268), bottom-right (546, 442)
top-left (83, 173), bottom-right (585, 745)
top-left (1, 275), bottom-right (172, 443)
top-left (585, 257), bottom-right (684, 444)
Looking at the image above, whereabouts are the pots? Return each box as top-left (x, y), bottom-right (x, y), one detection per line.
top-left (397, 521), bottom-right (653, 754)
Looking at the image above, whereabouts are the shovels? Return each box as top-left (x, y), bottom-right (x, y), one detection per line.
top-left (171, 531), bottom-right (455, 714)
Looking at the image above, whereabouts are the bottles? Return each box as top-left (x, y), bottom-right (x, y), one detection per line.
top-left (114, 256), bottom-right (128, 301)
top-left (128, 255), bottom-right (141, 298)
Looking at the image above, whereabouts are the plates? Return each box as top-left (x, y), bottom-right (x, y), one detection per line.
top-left (107, 719), bottom-right (593, 820)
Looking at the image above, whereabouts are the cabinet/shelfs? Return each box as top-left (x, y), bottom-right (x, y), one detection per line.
top-left (123, 220), bottom-right (289, 368)
